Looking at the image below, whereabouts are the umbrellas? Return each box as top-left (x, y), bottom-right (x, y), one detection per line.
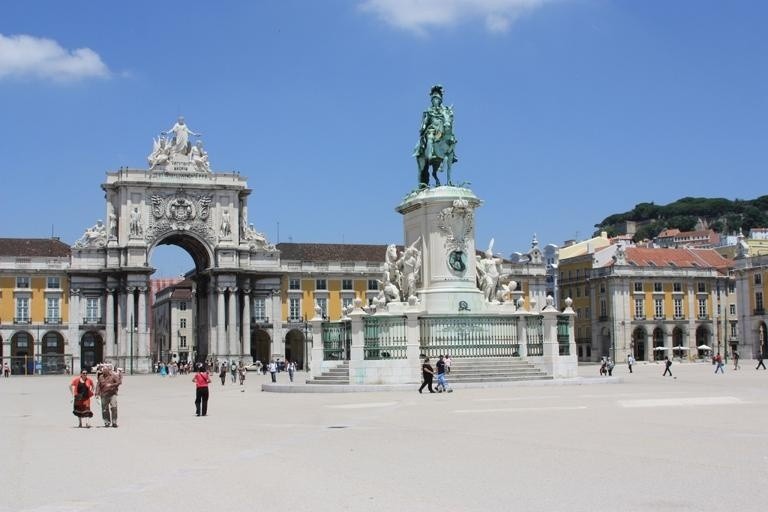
top-left (672, 346), bottom-right (690, 358)
top-left (651, 346), bottom-right (670, 356)
top-left (697, 344), bottom-right (712, 356)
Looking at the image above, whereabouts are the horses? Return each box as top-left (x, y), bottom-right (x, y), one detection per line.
top-left (382, 243), bottom-right (401, 286)
top-left (416, 103), bottom-right (454, 189)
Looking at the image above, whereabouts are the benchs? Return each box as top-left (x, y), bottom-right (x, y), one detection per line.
top-left (635, 357), bottom-right (712, 364)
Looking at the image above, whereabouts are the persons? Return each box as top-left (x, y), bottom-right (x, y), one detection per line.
top-left (69, 365), bottom-right (123, 428)
top-left (369, 235), bottom-right (518, 305)
top-left (703, 348), bottom-right (767, 374)
top-left (628, 355), bottom-right (636, 373)
top-left (599, 355), bottom-right (608, 376)
top-left (663, 356), bottom-right (673, 377)
top-left (606, 356), bottom-right (616, 376)
top-left (418, 84), bottom-right (459, 165)
top-left (72, 115), bottom-right (278, 252)
top-left (3, 362), bottom-right (10, 377)
top-left (158, 358), bottom-right (297, 417)
top-left (417, 353), bottom-right (452, 393)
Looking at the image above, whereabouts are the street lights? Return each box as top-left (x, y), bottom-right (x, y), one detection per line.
top-left (299, 311), bottom-right (313, 371)
top-left (125, 312), bottom-right (138, 374)
top-left (550, 263), bottom-right (558, 309)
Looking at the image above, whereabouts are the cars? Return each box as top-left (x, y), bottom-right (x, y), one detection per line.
top-left (266, 361), bottom-right (286, 371)
top-left (90, 363), bottom-right (124, 374)
top-left (245, 363), bottom-right (256, 371)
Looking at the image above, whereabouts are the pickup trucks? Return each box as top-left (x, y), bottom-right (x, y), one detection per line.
top-left (12, 361), bottom-right (25, 372)
